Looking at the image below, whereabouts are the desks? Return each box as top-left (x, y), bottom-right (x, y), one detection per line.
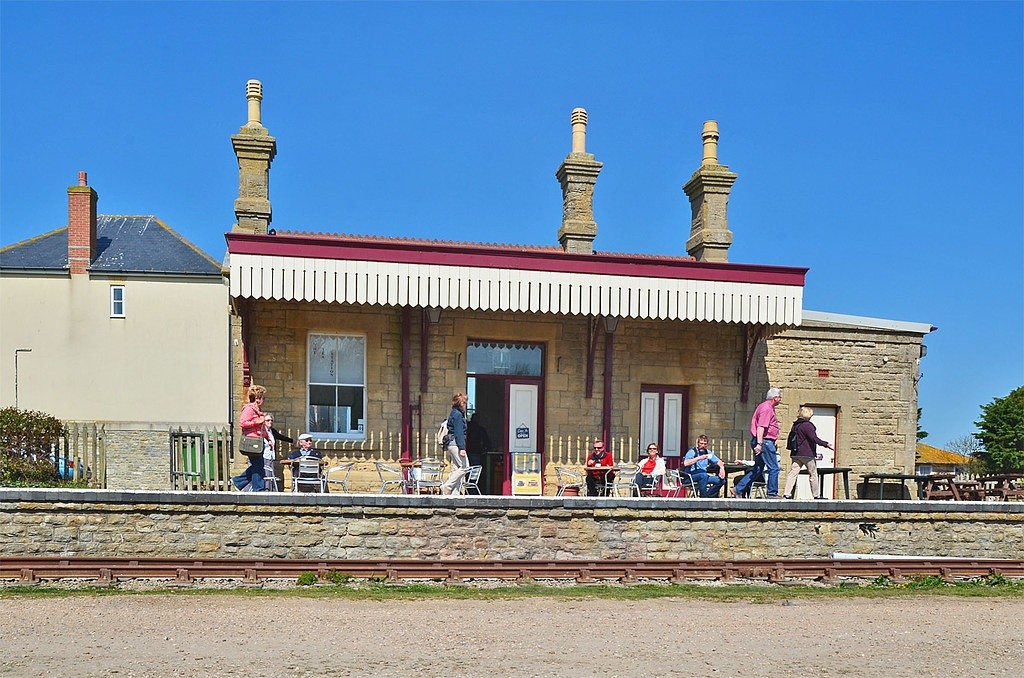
top-left (280, 459), bottom-right (328, 464)
top-left (914, 475), bottom-right (962, 502)
top-left (792, 468), bottom-right (852, 499)
top-left (722, 465), bottom-right (752, 499)
top-left (584, 465), bottom-right (620, 472)
top-left (993, 473), bottom-right (1024, 502)
top-left (859, 474), bottom-right (942, 501)
top-left (401, 462), bottom-right (448, 467)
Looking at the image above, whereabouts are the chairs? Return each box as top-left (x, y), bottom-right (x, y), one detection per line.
top-left (595, 458), bottom-right (663, 498)
top-left (247, 460), bottom-right (281, 492)
top-left (555, 467), bottom-right (587, 498)
top-left (450, 465), bottom-right (483, 496)
top-left (374, 461), bottom-right (408, 495)
top-left (730, 467), bottom-right (769, 499)
top-left (288, 455), bottom-right (324, 493)
top-left (322, 463), bottom-right (354, 493)
top-left (410, 458), bottom-right (447, 496)
top-left (666, 470), bottom-right (713, 498)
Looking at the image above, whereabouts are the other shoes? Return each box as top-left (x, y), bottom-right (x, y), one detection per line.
top-left (730, 487), bottom-right (742, 498)
top-left (230, 477), bottom-right (240, 491)
top-left (814, 497), bottom-right (828, 500)
top-left (783, 496), bottom-right (792, 499)
top-left (767, 495), bottom-right (782, 499)
top-left (440, 485), bottom-right (451, 495)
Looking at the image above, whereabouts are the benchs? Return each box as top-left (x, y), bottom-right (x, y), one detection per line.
top-left (933, 482), bottom-right (1024, 501)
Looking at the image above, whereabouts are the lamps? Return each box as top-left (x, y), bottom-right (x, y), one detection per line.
top-left (603, 317), bottom-right (620, 333)
top-left (426, 307), bottom-right (443, 326)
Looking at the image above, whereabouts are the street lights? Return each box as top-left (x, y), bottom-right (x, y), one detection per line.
top-left (14, 348), bottom-right (32, 411)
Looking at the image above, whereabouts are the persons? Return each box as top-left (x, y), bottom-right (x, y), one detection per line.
top-left (288, 434), bottom-right (326, 479)
top-left (585, 440), bottom-right (616, 497)
top-left (633, 442), bottom-right (666, 497)
top-left (730, 388), bottom-right (783, 499)
top-left (466, 412), bottom-right (490, 469)
top-left (783, 406), bottom-right (837, 499)
top-left (438, 392), bottom-right (469, 495)
top-left (733, 444), bottom-right (782, 499)
top-left (228, 384), bottom-right (273, 492)
top-left (682, 434), bottom-right (727, 498)
top-left (242, 413), bottom-right (296, 493)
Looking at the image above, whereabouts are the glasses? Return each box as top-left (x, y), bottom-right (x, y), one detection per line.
top-left (304, 439), bottom-right (312, 442)
top-left (649, 448), bottom-right (656, 450)
top-left (594, 447), bottom-right (602, 449)
top-left (265, 419), bottom-right (273, 422)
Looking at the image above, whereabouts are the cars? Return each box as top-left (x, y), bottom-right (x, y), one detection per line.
top-left (8, 446), bottom-right (92, 481)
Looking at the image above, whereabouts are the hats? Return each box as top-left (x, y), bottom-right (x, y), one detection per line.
top-left (299, 434), bottom-right (313, 439)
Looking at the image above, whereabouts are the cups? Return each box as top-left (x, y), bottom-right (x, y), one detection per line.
top-left (706, 454), bottom-right (712, 459)
top-left (595, 464), bottom-right (601, 468)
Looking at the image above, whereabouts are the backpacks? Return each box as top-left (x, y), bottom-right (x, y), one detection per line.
top-left (787, 422), bottom-right (807, 450)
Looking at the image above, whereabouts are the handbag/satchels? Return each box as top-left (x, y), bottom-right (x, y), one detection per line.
top-left (240, 436), bottom-right (265, 454)
